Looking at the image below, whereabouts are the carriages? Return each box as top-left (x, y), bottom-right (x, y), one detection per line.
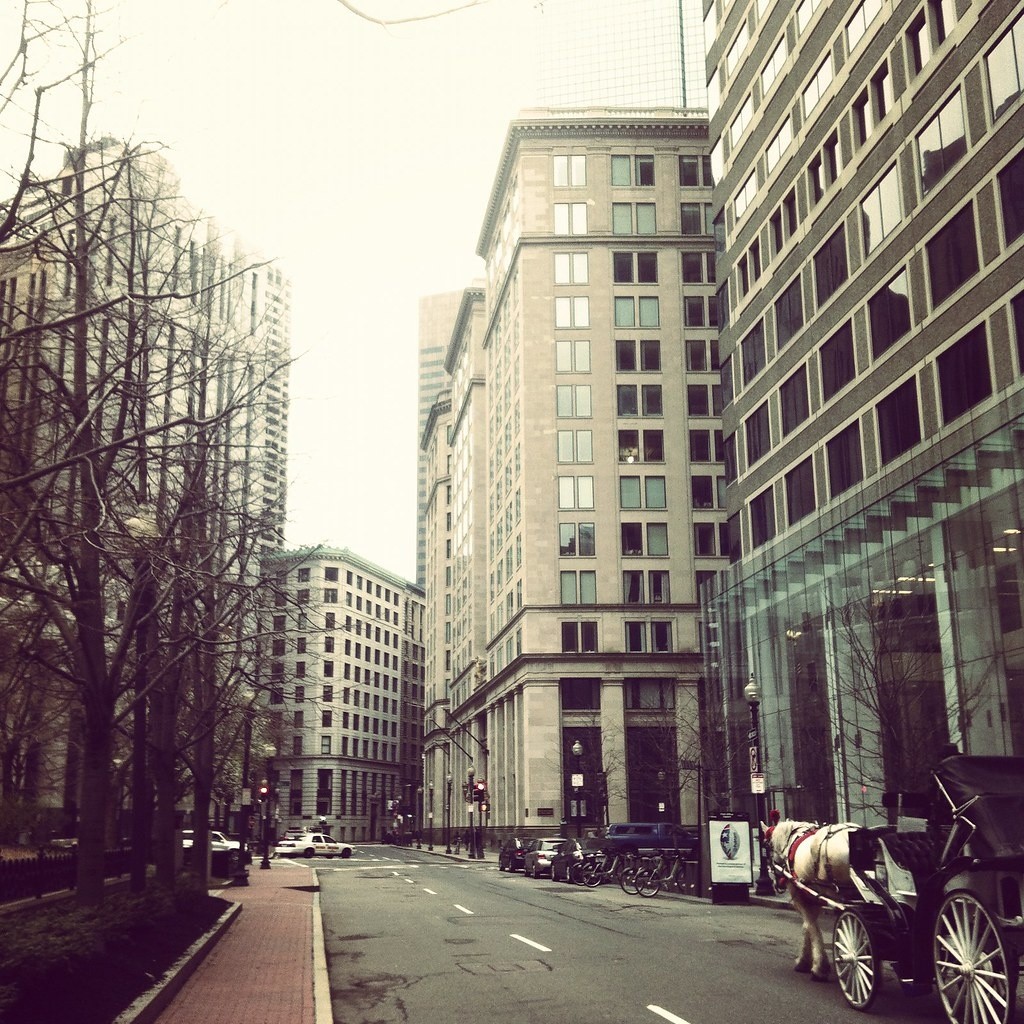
top-left (758, 744), bottom-right (1024, 1023)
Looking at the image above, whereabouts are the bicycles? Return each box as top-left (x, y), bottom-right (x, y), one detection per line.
top-left (571, 848), bottom-right (692, 897)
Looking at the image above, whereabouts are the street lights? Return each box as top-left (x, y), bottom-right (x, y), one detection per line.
top-left (468, 767), bottom-right (478, 859)
top-left (258, 779), bottom-right (272, 870)
top-left (570, 739), bottom-right (583, 836)
top-left (445, 775), bottom-right (453, 854)
top-left (415, 785), bottom-right (423, 849)
top-left (743, 670), bottom-right (765, 827)
top-left (427, 780), bottom-right (434, 851)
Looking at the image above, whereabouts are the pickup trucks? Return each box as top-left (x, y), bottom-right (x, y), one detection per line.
top-left (586, 823), bottom-right (699, 850)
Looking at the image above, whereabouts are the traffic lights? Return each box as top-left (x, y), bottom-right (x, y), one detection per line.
top-left (479, 804), bottom-right (490, 811)
top-left (478, 783), bottom-right (484, 802)
top-left (473, 783), bottom-right (479, 802)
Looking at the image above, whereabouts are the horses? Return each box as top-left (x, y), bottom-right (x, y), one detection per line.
top-left (757, 818), bottom-right (889, 985)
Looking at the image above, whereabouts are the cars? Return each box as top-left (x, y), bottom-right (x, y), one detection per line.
top-left (550, 836), bottom-right (619, 885)
top-left (181, 830), bottom-right (249, 853)
top-left (47, 830), bottom-right (80, 848)
top-left (278, 832), bottom-right (358, 859)
top-left (523, 837), bottom-right (584, 879)
top-left (498, 837), bottom-right (536, 873)
